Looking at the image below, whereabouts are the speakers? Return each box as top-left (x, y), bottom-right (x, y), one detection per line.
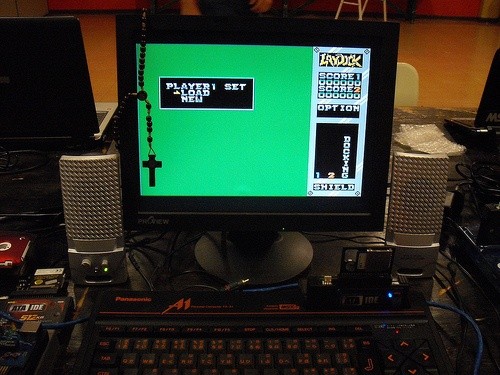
top-left (385, 152), bottom-right (450, 278)
top-left (59, 153), bottom-right (128, 285)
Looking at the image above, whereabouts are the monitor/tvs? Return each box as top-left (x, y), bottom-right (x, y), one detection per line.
top-left (115, 14), bottom-right (400, 284)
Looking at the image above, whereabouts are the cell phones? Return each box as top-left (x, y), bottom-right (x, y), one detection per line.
top-left (0, 234), bottom-right (31, 267)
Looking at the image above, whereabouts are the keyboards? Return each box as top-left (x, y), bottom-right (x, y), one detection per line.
top-left (75, 289), bottom-right (454, 375)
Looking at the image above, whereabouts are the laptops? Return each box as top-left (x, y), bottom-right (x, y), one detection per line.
top-left (445, 48), bottom-right (500, 135)
top-left (0, 15), bottom-right (118, 145)
